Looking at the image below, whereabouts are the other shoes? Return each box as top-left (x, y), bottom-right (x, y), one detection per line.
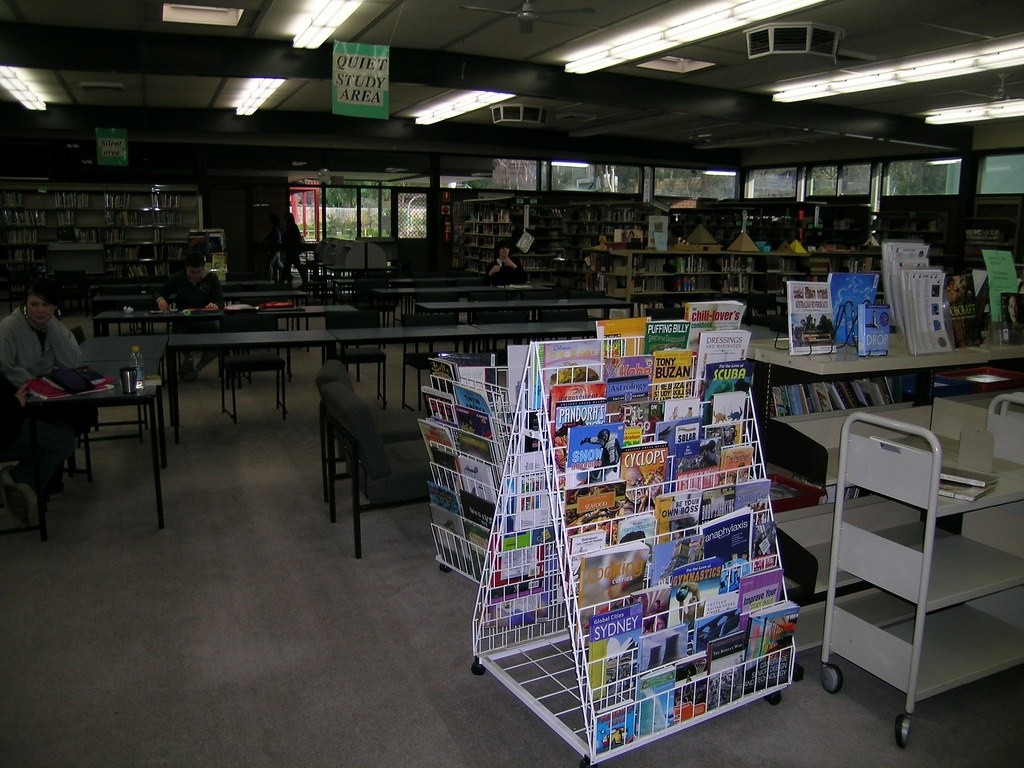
top-left (181, 358), bottom-right (199, 381)
top-left (0, 464), bottom-right (16, 485)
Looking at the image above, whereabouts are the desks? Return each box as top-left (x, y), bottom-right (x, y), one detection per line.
top-left (25, 273), bottom-right (637, 551)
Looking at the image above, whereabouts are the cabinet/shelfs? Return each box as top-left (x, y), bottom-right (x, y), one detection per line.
top-left (0, 183), bottom-right (204, 311)
top-left (423, 197), bottom-right (1024, 746)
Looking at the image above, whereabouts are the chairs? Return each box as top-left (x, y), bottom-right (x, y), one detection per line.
top-left (218, 315), bottom-right (289, 424)
top-left (473, 311), bottom-right (529, 367)
top-left (398, 314), bottom-right (458, 413)
top-left (537, 309), bottom-right (590, 344)
top-left (321, 388), bottom-right (432, 557)
top-left (324, 310), bottom-right (388, 410)
top-left (316, 358), bottom-right (425, 504)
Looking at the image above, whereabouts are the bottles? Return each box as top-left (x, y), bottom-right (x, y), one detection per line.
top-left (129, 346), bottom-right (144, 391)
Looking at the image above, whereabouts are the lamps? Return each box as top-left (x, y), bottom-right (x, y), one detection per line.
top-left (237, 0), bottom-right (1024, 127)
top-left (0, 65), bottom-right (46, 113)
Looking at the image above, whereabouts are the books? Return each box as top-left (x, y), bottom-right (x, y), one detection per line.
top-left (787, 280), bottom-right (837, 355)
top-left (26, 366), bottom-right (115, 400)
top-left (672, 213), bottom-right (1008, 317)
top-left (939, 466), bottom-right (999, 502)
top-left (260, 302), bottom-right (297, 311)
top-left (0, 191), bottom-right (227, 282)
top-left (858, 304), bottom-right (891, 356)
top-left (144, 375), bottom-right (161, 385)
top-left (532, 301), bottom-right (801, 753)
top-left (820, 485), bottom-right (860, 503)
top-left (463, 203), bottom-right (669, 319)
top-left (768, 376), bottom-right (895, 418)
top-left (417, 357), bottom-right (567, 626)
top-left (828, 272), bottom-right (879, 344)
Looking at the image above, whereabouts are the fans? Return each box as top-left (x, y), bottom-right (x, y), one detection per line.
top-left (460, 0), bottom-right (602, 34)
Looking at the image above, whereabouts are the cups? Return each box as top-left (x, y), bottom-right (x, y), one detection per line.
top-left (120, 367), bottom-right (137, 394)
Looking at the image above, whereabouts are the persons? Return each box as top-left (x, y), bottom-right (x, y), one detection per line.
top-left (577, 429), bottom-right (621, 485)
top-left (265, 214), bottom-right (307, 289)
top-left (590, 226), bottom-right (606, 248)
top-left (154, 252), bottom-right (225, 380)
top-left (0, 284), bottom-right (98, 528)
top-left (486, 241), bottom-right (529, 285)
